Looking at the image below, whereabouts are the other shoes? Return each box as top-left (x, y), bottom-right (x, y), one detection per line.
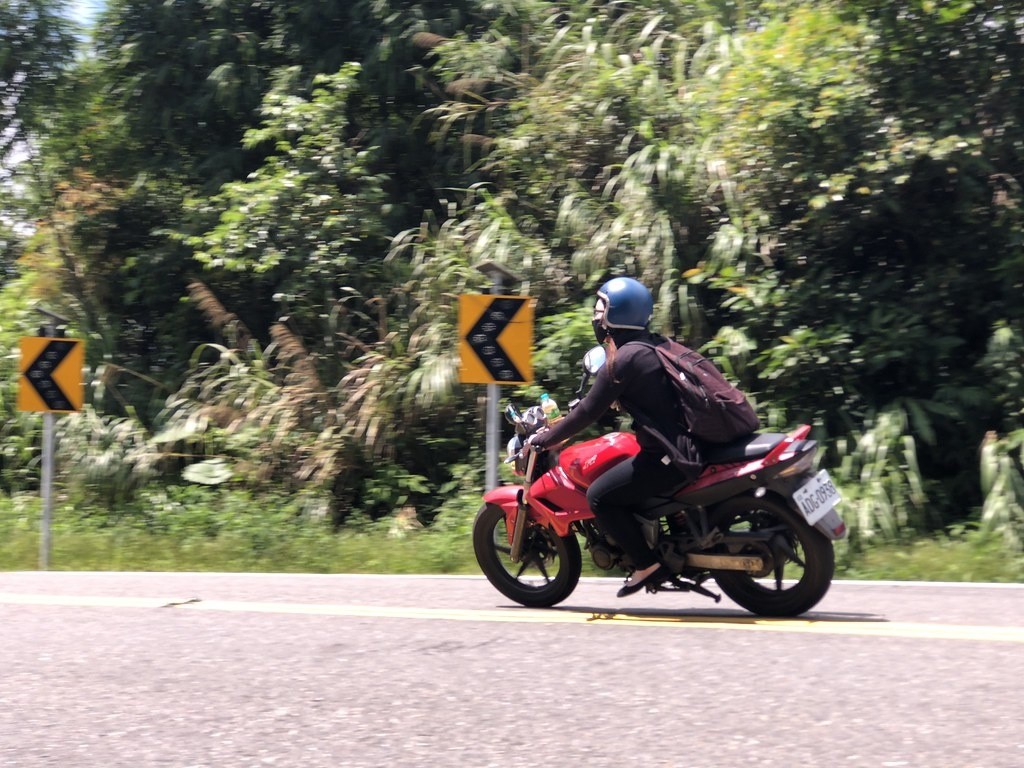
top-left (617, 563), bottom-right (665, 598)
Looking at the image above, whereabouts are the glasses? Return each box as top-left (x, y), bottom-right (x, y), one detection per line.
top-left (594, 308), bottom-right (604, 314)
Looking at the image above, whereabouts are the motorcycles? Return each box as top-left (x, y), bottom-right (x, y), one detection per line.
top-left (472, 347), bottom-right (847, 620)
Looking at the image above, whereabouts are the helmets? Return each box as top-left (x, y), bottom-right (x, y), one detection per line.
top-left (597, 276), bottom-right (653, 330)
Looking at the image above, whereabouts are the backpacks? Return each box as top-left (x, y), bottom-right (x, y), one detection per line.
top-left (620, 332), bottom-right (760, 445)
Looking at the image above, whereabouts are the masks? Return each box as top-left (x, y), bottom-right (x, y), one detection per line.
top-left (592, 319), bottom-right (614, 344)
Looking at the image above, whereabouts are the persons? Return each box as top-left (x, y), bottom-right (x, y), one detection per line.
top-left (520, 275), bottom-right (712, 598)
